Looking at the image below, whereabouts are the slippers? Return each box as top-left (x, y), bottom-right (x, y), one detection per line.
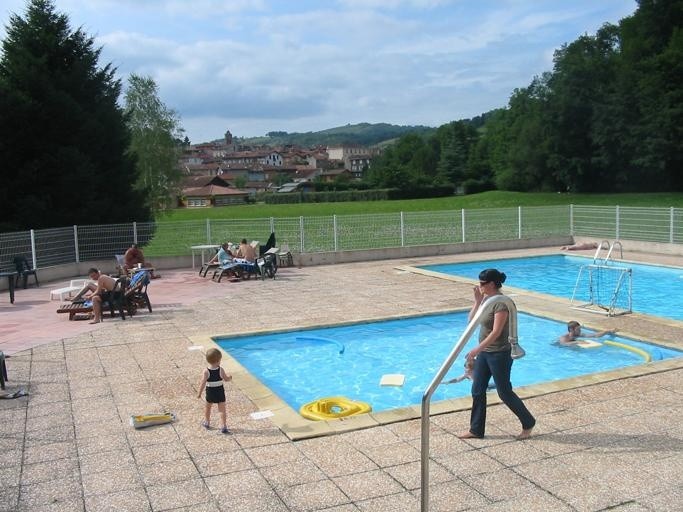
top-left (0, 389), bottom-right (27, 399)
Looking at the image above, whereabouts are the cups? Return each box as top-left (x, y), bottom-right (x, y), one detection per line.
top-left (137, 262), bottom-right (142, 268)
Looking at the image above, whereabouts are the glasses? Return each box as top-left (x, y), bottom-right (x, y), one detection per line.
top-left (480, 280), bottom-right (491, 285)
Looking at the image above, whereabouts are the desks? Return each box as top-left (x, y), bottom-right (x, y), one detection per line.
top-left (190, 245), bottom-right (220, 270)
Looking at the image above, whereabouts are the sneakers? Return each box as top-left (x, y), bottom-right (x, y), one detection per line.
top-left (221, 425), bottom-right (228, 432)
top-left (201, 420), bottom-right (209, 427)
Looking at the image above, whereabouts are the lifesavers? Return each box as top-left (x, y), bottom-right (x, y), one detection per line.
top-left (299, 395), bottom-right (371, 422)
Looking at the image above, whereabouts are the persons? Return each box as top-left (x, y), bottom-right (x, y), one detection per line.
top-left (557, 317), bottom-right (620, 350)
top-left (217, 241), bottom-right (238, 278)
top-left (232, 237), bottom-right (259, 264)
top-left (193, 348), bottom-right (233, 436)
top-left (63, 274), bottom-right (130, 303)
top-left (120, 242), bottom-right (160, 280)
top-left (458, 266), bottom-right (536, 442)
top-left (208, 240), bottom-right (242, 265)
top-left (86, 266), bottom-right (120, 325)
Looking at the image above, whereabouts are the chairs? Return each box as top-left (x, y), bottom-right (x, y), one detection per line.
top-left (0, 351), bottom-right (8, 390)
top-left (0, 233), bottom-right (155, 320)
top-left (198, 240), bottom-right (293, 283)
